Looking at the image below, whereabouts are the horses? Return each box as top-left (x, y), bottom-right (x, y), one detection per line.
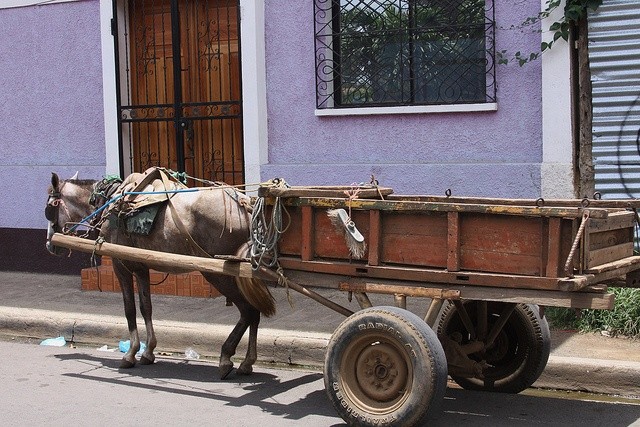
top-left (45, 171), bottom-right (276, 380)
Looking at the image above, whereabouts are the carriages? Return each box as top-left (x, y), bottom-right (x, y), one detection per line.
top-left (45, 167), bottom-right (640, 427)
